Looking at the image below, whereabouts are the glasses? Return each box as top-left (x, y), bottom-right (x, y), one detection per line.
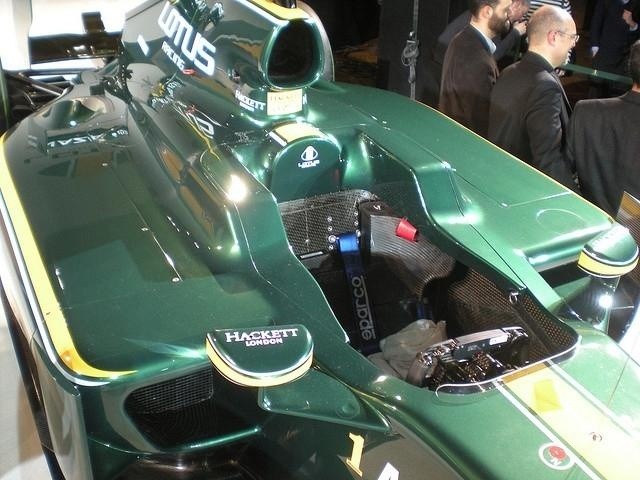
top-left (557, 31), bottom-right (580, 42)
